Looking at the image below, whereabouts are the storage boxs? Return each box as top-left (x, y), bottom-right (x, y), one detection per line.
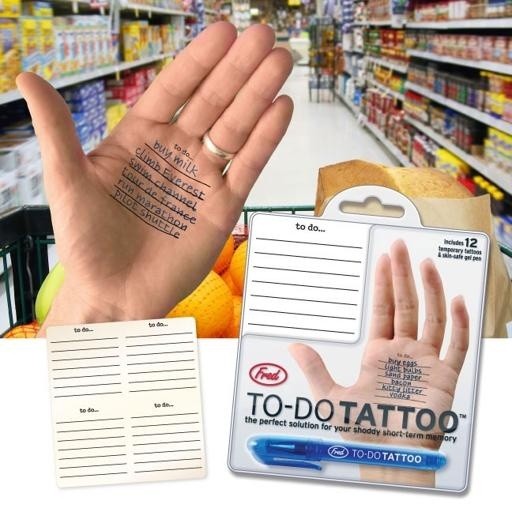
top-left (0, 135), bottom-right (47, 274)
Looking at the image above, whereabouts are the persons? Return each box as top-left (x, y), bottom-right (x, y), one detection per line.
top-left (17, 19), bottom-right (294, 338)
top-left (291, 239), bottom-right (469, 486)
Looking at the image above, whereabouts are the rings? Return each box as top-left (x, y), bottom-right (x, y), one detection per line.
top-left (201, 131), bottom-right (234, 163)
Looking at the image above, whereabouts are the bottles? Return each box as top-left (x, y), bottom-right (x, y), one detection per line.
top-left (432, 65), bottom-right (489, 111)
top-left (449, 116), bottom-right (475, 157)
top-left (474, 174), bottom-right (503, 219)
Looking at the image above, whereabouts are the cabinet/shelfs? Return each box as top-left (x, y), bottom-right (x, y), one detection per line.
top-left (336, 1), bottom-right (511, 255)
top-left (1, 0), bottom-right (224, 281)
